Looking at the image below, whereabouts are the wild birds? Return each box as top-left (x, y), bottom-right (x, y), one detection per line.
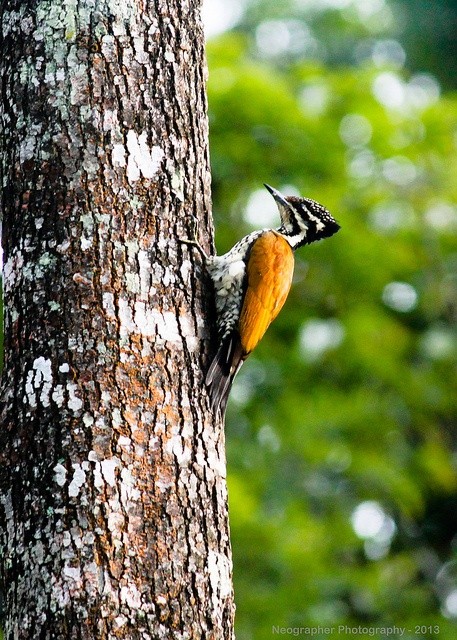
top-left (176, 180), bottom-right (343, 425)
